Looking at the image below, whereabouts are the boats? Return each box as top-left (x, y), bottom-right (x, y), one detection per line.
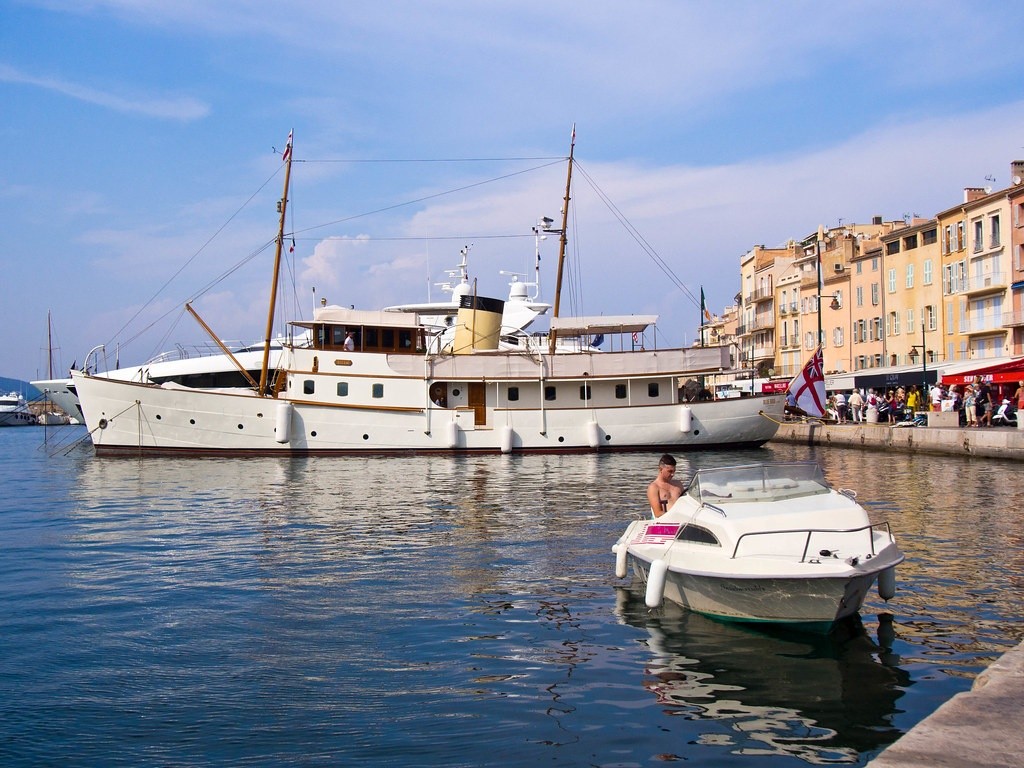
top-left (611, 460), bottom-right (907, 629)
top-left (0, 389), bottom-right (35, 427)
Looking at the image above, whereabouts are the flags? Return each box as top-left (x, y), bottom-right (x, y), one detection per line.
top-left (593, 334), bottom-right (603, 346)
top-left (633, 333), bottom-right (638, 342)
top-left (702, 291), bottom-right (710, 319)
top-left (69, 362), bottom-right (75, 373)
top-left (789, 347), bottom-right (825, 416)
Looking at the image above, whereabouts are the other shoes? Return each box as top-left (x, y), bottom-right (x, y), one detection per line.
top-left (987, 424), bottom-right (993, 427)
top-left (979, 418), bottom-right (984, 424)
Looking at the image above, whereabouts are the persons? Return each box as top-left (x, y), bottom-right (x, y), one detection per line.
top-left (897, 385), bottom-right (922, 421)
top-left (885, 390), bottom-right (895, 424)
top-left (847, 389), bottom-right (864, 424)
top-left (832, 390), bottom-right (847, 424)
top-left (344, 332), bottom-right (355, 351)
top-left (647, 455), bottom-right (684, 517)
top-left (828, 400), bottom-right (839, 419)
top-left (981, 381), bottom-right (994, 427)
top-left (786, 392), bottom-right (795, 407)
top-left (1015, 381), bottom-right (1024, 409)
top-left (865, 388), bottom-right (882, 411)
top-left (964, 374), bottom-right (987, 428)
top-left (926, 382), bottom-right (961, 411)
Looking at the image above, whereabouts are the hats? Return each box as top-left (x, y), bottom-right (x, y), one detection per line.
top-left (965, 385), bottom-right (972, 388)
top-left (888, 390), bottom-right (894, 393)
top-left (853, 389), bottom-right (858, 392)
top-left (936, 382), bottom-right (940, 385)
top-left (836, 390), bottom-right (840, 393)
top-left (1019, 380), bottom-right (1024, 386)
top-left (985, 380), bottom-right (991, 384)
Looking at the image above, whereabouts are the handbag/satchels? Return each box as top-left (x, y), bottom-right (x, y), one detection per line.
top-left (941, 399), bottom-right (954, 412)
top-left (971, 398), bottom-right (976, 404)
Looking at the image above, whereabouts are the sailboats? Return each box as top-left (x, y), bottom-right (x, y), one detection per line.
top-left (29, 306), bottom-right (67, 427)
top-left (70, 122), bottom-right (792, 463)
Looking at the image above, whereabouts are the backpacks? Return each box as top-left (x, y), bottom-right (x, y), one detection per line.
top-left (869, 394), bottom-right (877, 405)
top-left (893, 400), bottom-right (897, 409)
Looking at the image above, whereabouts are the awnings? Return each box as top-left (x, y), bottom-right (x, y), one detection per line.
top-left (826, 357), bottom-right (1024, 389)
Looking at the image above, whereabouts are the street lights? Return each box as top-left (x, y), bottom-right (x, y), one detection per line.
top-left (814, 241), bottom-right (841, 374)
top-left (910, 321), bottom-right (933, 409)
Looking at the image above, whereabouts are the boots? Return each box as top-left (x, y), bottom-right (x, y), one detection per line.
top-left (965, 421), bottom-right (971, 428)
top-left (972, 421), bottom-right (978, 426)
